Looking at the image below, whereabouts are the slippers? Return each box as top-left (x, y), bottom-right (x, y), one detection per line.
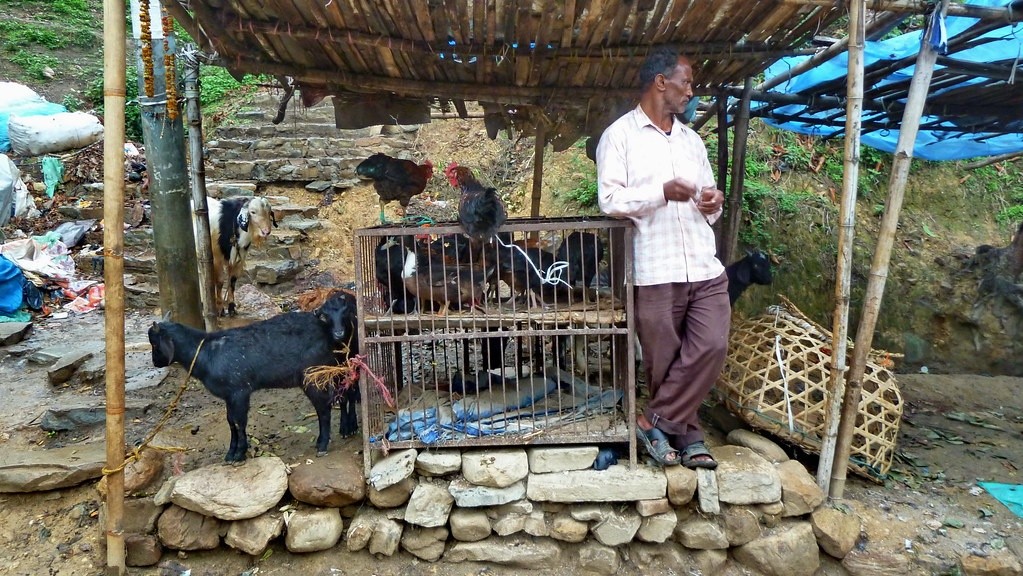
top-left (636, 421), bottom-right (682, 466)
top-left (680, 441), bottom-right (718, 467)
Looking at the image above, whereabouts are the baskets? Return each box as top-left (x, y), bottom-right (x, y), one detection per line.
top-left (716, 293), bottom-right (906, 484)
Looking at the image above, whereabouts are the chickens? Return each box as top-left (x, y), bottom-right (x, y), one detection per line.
top-left (354, 152), bottom-right (434, 227)
top-left (445, 162), bottom-right (506, 241)
top-left (418, 213), bottom-right (603, 316)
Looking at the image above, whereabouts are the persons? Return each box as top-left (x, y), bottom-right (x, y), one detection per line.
top-left (594, 47), bottom-right (731, 470)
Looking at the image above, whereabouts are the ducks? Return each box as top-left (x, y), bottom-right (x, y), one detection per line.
top-left (374, 233), bottom-right (496, 317)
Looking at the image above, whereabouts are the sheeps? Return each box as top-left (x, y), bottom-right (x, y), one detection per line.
top-left (631, 249), bottom-right (772, 399)
top-left (148, 312), bottom-right (335, 466)
top-left (190, 195), bottom-right (278, 321)
top-left (317, 291), bottom-right (360, 440)
